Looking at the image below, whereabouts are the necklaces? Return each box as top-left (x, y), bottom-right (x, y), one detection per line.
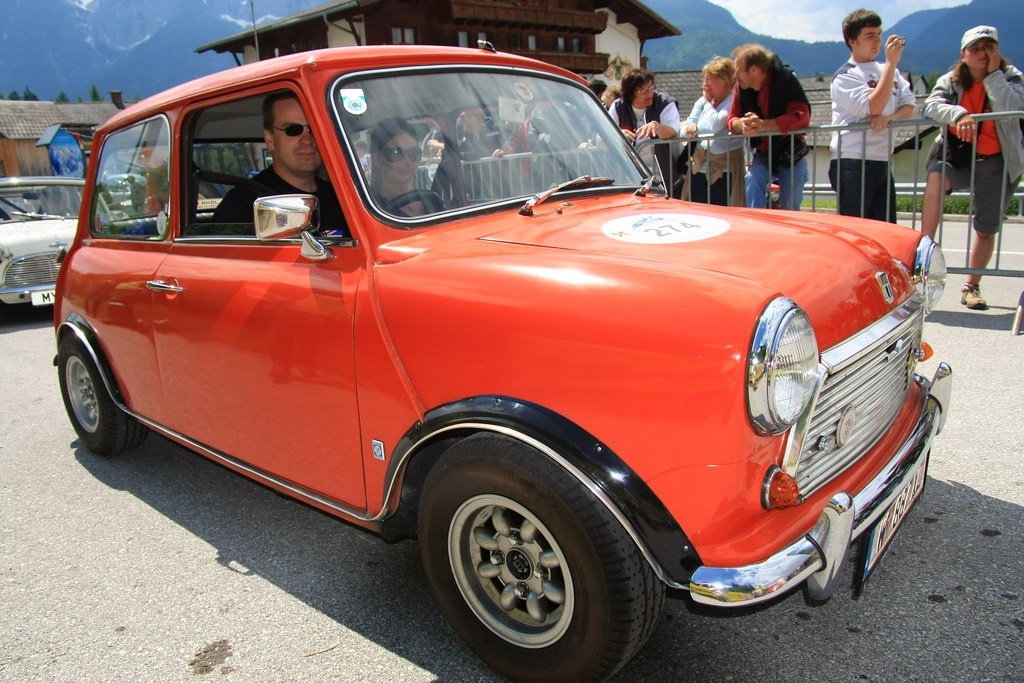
top-left (408, 204), bottom-right (414, 212)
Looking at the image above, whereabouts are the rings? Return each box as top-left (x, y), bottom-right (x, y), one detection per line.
top-left (967, 126), bottom-right (971, 128)
top-left (961, 126), bottom-right (966, 129)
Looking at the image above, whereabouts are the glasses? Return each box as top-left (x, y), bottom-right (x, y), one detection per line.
top-left (380, 148), bottom-right (422, 162)
top-left (272, 124), bottom-right (312, 137)
top-left (635, 84), bottom-right (655, 93)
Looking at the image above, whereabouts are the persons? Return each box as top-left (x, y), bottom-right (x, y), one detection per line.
top-left (829, 8), bottom-right (916, 225)
top-left (421, 99), bottom-right (577, 178)
top-left (596, 69), bottom-right (684, 200)
top-left (353, 140), bottom-right (372, 168)
top-left (575, 79), bottom-right (622, 152)
top-left (680, 57), bottom-right (747, 208)
top-left (752, 356), bottom-right (758, 392)
top-left (370, 117), bottom-right (425, 218)
top-left (210, 90), bottom-right (351, 238)
top-left (725, 43), bottom-right (812, 210)
top-left (920, 25), bottom-right (1024, 309)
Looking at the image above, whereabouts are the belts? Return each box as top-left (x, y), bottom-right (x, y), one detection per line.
top-left (976, 152), bottom-right (1002, 161)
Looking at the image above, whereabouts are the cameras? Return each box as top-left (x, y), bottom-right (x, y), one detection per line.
top-left (898, 36), bottom-right (906, 45)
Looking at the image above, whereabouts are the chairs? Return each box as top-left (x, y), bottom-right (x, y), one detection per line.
top-left (38, 187), bottom-right (72, 217)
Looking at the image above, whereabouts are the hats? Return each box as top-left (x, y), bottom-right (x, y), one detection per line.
top-left (960, 25), bottom-right (998, 50)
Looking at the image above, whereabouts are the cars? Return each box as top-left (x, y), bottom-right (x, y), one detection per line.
top-left (50, 45), bottom-right (955, 678)
top-left (105, 173), bottom-right (155, 209)
top-left (0, 177), bottom-right (115, 307)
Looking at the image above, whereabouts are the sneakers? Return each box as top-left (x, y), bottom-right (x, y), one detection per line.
top-left (961, 287), bottom-right (987, 309)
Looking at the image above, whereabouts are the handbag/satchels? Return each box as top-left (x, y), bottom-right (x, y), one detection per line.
top-left (947, 130), bottom-right (972, 168)
top-left (676, 141), bottom-right (698, 175)
top-left (772, 135), bottom-right (809, 168)
